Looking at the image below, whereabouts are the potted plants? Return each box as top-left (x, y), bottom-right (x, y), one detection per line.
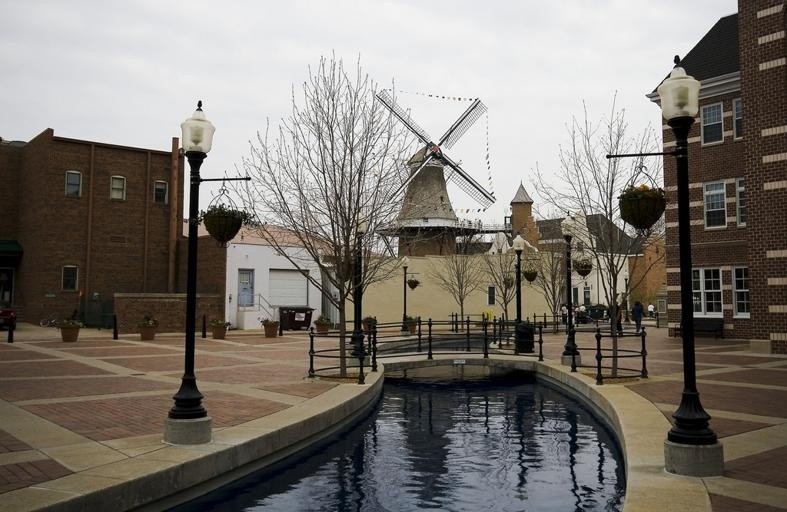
top-left (58, 319), bottom-right (82, 343)
top-left (188, 201), bottom-right (270, 246)
top-left (405, 278), bottom-right (421, 290)
top-left (361, 315), bottom-right (378, 335)
top-left (133, 317), bottom-right (160, 341)
top-left (205, 317), bottom-right (233, 340)
top-left (313, 312), bottom-right (332, 337)
top-left (258, 318), bottom-right (281, 338)
top-left (571, 258), bottom-right (592, 279)
top-left (521, 269), bottom-right (538, 285)
top-left (404, 314), bottom-right (420, 334)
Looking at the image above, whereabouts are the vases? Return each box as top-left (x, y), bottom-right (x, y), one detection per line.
top-left (617, 200), bottom-right (667, 231)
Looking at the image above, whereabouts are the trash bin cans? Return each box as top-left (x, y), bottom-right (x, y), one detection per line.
top-left (517, 322), bottom-right (534, 353)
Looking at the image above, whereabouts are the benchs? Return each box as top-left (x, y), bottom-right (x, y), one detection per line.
top-left (670, 315), bottom-right (725, 340)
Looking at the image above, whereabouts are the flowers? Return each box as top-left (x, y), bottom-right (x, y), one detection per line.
top-left (618, 183), bottom-right (665, 199)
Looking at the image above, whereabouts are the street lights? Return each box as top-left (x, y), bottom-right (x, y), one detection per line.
top-left (622, 269), bottom-right (630, 325)
top-left (558, 212), bottom-right (597, 364)
top-left (164, 99), bottom-right (253, 443)
top-left (511, 230), bottom-right (539, 325)
top-left (399, 256), bottom-right (419, 338)
top-left (606, 53), bottom-right (730, 473)
top-left (347, 212), bottom-right (369, 366)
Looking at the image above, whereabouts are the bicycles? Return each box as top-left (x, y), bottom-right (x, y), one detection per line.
top-left (38, 313), bottom-right (60, 328)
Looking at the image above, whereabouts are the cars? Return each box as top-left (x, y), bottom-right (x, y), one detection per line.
top-left (0, 301), bottom-right (16, 329)
top-left (579, 303), bottom-right (609, 321)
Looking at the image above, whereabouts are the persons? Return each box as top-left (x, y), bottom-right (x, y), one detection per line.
top-left (607, 302), bottom-right (623, 336)
top-left (562, 303), bottom-right (586, 323)
top-left (647, 302), bottom-right (654, 319)
top-left (631, 301), bottom-right (647, 332)
